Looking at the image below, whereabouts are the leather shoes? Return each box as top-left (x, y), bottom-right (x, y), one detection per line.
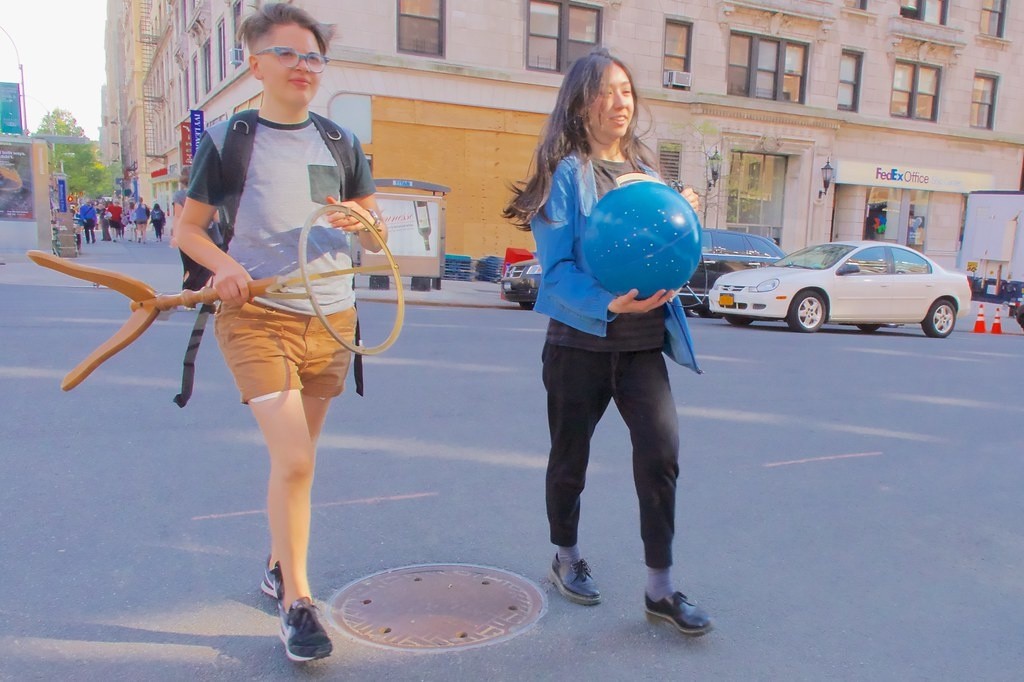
top-left (645, 587), bottom-right (712, 636)
top-left (550, 552), bottom-right (601, 605)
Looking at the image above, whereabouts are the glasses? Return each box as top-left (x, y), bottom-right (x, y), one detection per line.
top-left (257, 46), bottom-right (331, 73)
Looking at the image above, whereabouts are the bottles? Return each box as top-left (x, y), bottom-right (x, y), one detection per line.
top-left (413, 201), bottom-right (431, 251)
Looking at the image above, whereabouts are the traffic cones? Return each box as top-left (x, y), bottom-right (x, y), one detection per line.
top-left (971, 304), bottom-right (988, 334)
top-left (989, 308), bottom-right (1010, 335)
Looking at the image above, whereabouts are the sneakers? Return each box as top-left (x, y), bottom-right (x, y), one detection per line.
top-left (278, 598), bottom-right (334, 662)
top-left (260, 553), bottom-right (282, 598)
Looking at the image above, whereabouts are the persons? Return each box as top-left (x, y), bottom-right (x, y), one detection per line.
top-left (170, 2), bottom-right (389, 662)
top-left (498, 50), bottom-right (714, 635)
top-left (79, 196), bottom-right (166, 243)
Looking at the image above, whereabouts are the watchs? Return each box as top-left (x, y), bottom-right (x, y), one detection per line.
top-left (362, 209), bottom-right (380, 233)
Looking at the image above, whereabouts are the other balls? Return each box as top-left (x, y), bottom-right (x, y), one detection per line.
top-left (582, 182), bottom-right (704, 296)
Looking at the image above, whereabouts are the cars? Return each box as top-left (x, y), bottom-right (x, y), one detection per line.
top-left (707, 242), bottom-right (973, 339)
top-left (499, 228), bottom-right (792, 319)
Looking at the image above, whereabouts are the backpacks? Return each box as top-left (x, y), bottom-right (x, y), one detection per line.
top-left (136, 203), bottom-right (147, 224)
top-left (153, 209), bottom-right (162, 221)
top-left (170, 109), bottom-right (355, 290)
